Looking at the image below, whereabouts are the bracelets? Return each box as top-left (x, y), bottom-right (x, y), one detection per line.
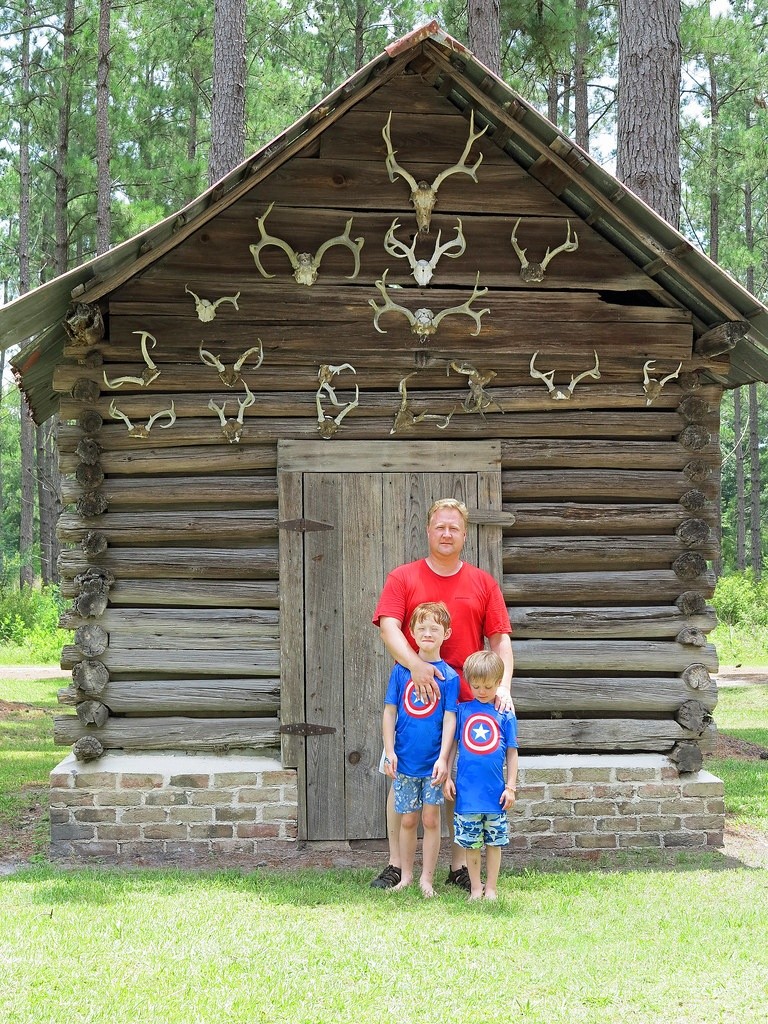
top-left (505, 784), bottom-right (517, 793)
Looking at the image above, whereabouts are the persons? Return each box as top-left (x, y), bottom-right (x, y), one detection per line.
top-left (371, 498), bottom-right (516, 897)
top-left (441, 649), bottom-right (519, 902)
top-left (381, 600), bottom-right (460, 897)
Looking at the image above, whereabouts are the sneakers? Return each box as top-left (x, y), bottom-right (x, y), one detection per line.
top-left (371, 864), bottom-right (401, 890)
top-left (445, 864), bottom-right (486, 895)
